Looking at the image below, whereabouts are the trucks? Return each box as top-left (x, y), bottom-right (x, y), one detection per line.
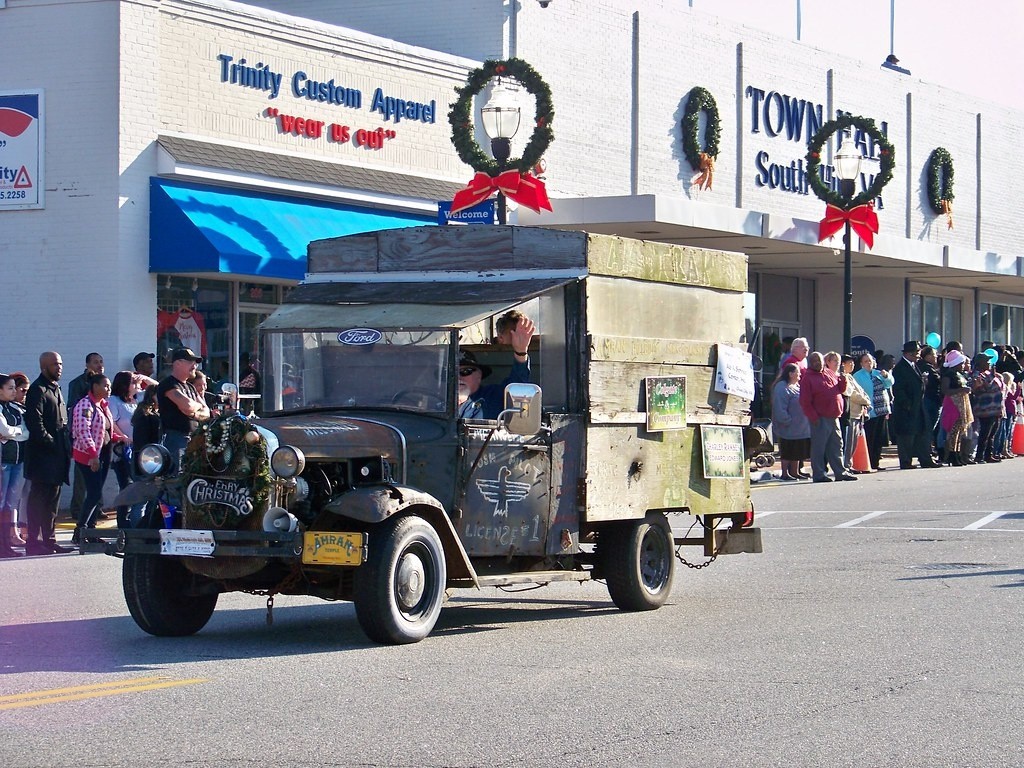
top-left (79, 224), bottom-right (761, 649)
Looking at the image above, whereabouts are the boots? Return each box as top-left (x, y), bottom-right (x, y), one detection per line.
top-left (0, 509), bottom-right (23, 558)
top-left (20, 527), bottom-right (28, 541)
top-left (10, 509), bottom-right (26, 547)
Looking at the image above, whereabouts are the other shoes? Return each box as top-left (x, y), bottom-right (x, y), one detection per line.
top-left (792, 474), bottom-right (808, 480)
top-left (98, 512), bottom-right (109, 519)
top-left (71, 529), bottom-right (89, 546)
top-left (835, 475), bottom-right (856, 481)
top-left (849, 440), bottom-right (1018, 474)
top-left (48, 543), bottom-right (74, 553)
top-left (26, 542), bottom-right (57, 555)
top-left (813, 477), bottom-right (832, 483)
top-left (87, 535), bottom-right (106, 543)
top-left (781, 475), bottom-right (797, 481)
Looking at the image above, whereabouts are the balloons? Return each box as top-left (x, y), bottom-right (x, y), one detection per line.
top-left (927, 333), bottom-right (940, 348)
top-left (985, 350), bottom-right (998, 365)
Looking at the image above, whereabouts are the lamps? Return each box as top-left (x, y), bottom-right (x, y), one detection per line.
top-left (192, 278), bottom-right (198, 291)
top-left (165, 275), bottom-right (171, 289)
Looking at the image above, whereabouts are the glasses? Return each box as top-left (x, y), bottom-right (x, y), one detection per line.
top-left (459, 367), bottom-right (477, 376)
top-left (15, 387), bottom-right (29, 393)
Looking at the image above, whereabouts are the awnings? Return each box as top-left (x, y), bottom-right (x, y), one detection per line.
top-left (149, 178), bottom-right (439, 281)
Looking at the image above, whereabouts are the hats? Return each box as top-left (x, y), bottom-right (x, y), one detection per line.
top-left (172, 349), bottom-right (203, 364)
top-left (943, 349), bottom-right (967, 368)
top-left (901, 340), bottom-right (923, 353)
top-left (973, 354), bottom-right (994, 363)
top-left (434, 349), bottom-right (493, 381)
top-left (133, 352), bottom-right (155, 367)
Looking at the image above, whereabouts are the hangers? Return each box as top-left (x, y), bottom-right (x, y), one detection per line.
top-left (177, 304), bottom-right (193, 313)
top-left (157, 305), bottom-right (163, 312)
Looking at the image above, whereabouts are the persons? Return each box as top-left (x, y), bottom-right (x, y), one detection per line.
top-left (159, 349), bottom-right (209, 473)
top-left (490, 310), bottom-right (527, 344)
top-left (69, 353), bottom-right (109, 519)
top-left (0, 373), bottom-right (30, 557)
top-left (22, 351), bottom-right (75, 556)
top-left (71, 371), bottom-right (130, 544)
top-left (892, 341), bottom-right (1024, 469)
top-left (772, 337), bottom-right (895, 483)
top-left (108, 352), bottom-right (160, 528)
top-left (427, 315), bottom-right (535, 420)
top-left (191, 372), bottom-right (207, 401)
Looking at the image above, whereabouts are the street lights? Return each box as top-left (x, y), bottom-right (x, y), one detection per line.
top-left (831, 126), bottom-right (863, 357)
top-left (481, 74), bottom-right (525, 224)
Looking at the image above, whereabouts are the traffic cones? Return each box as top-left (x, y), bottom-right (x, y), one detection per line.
top-left (852, 427), bottom-right (879, 474)
top-left (1010, 401), bottom-right (1024, 457)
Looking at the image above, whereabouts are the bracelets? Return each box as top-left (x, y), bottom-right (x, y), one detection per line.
top-left (514, 351), bottom-right (529, 356)
top-left (189, 412), bottom-right (199, 420)
